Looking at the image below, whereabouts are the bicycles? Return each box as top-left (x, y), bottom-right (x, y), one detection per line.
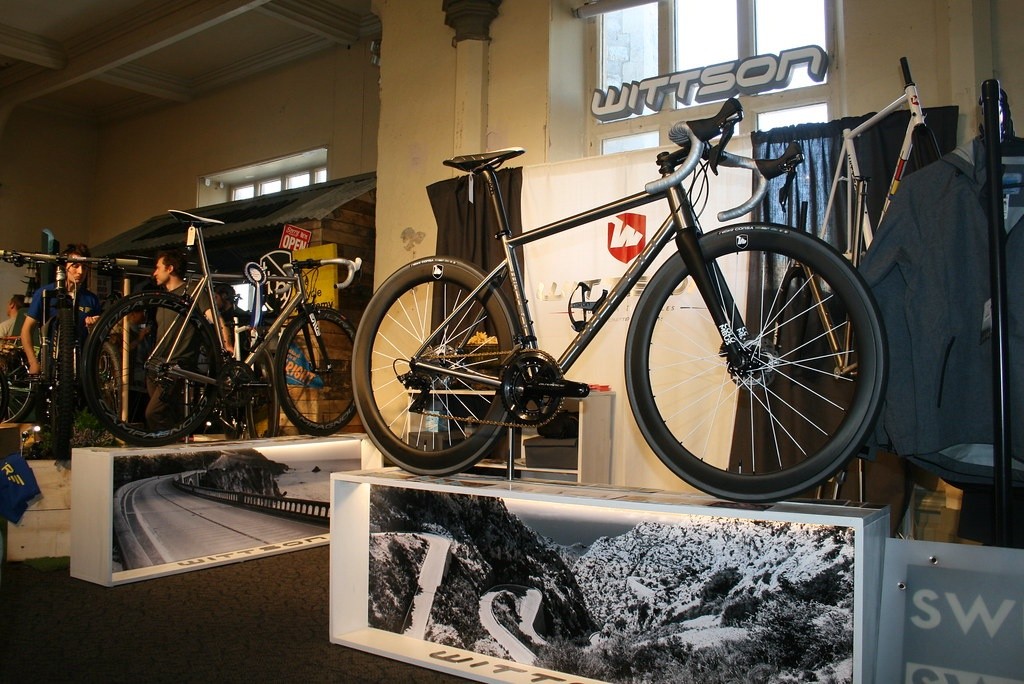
top-left (0, 245), bottom-right (152, 461)
top-left (78, 205), bottom-right (368, 448)
top-left (349, 96), bottom-right (889, 506)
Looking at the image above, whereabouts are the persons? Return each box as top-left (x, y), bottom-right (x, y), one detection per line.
top-left (0, 238), bottom-right (251, 446)
